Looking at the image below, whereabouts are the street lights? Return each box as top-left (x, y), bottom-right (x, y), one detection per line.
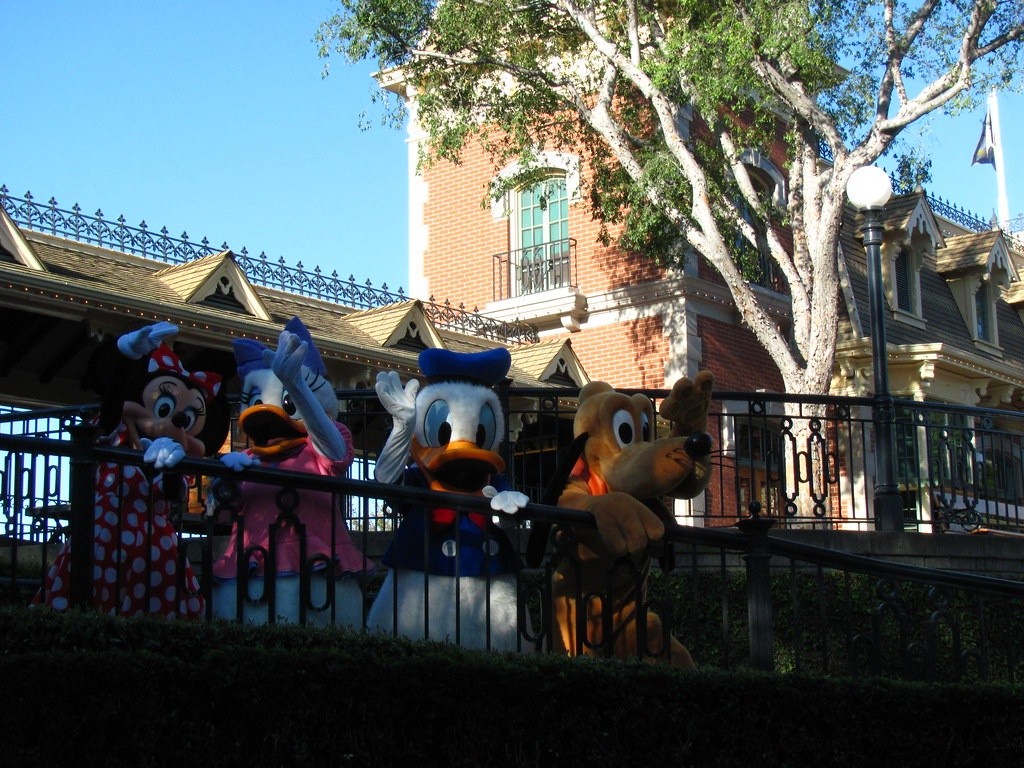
top-left (847, 164), bottom-right (903, 531)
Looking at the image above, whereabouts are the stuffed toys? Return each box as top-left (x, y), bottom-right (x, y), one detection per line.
top-left (366, 348), bottom-right (534, 652)
top-left (30, 321), bottom-right (220, 620)
top-left (543, 371), bottom-right (714, 669)
top-left (206, 315), bottom-right (376, 634)
